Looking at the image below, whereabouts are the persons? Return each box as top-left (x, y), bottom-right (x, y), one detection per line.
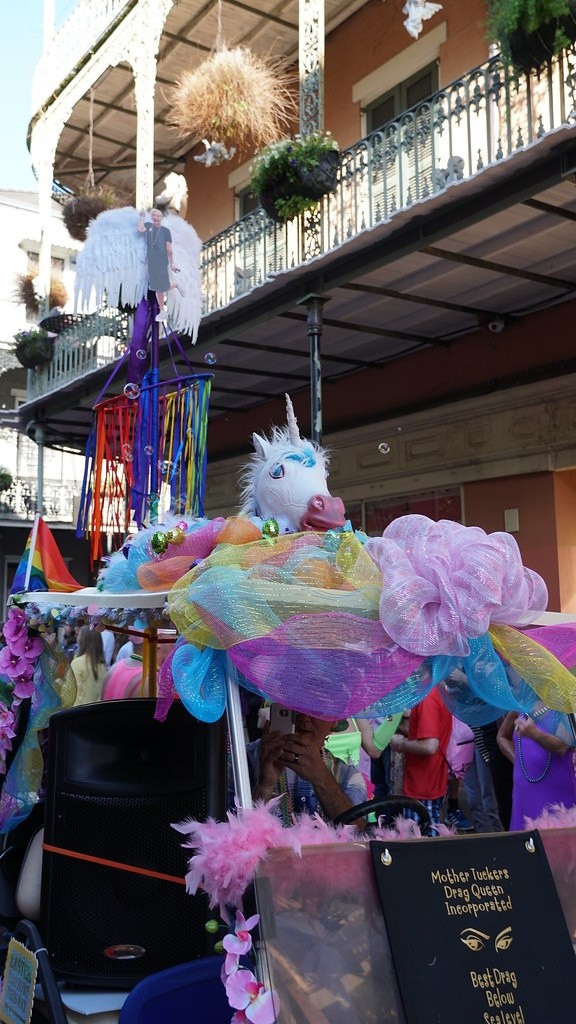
top-left (45, 616), bottom-right (178, 707)
top-left (138, 205), bottom-right (187, 323)
top-left (161, 172), bottom-right (190, 225)
top-left (324, 656), bottom-right (576, 833)
top-left (226, 667), bottom-right (369, 835)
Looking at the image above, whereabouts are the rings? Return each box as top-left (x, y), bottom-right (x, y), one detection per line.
top-left (294, 755), bottom-right (299, 763)
top-left (516, 725), bottom-right (519, 730)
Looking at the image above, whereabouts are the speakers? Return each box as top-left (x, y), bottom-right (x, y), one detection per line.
top-left (39, 697), bottom-right (231, 991)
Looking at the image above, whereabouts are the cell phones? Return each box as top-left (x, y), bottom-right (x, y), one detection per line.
top-left (269, 704), bottom-right (296, 738)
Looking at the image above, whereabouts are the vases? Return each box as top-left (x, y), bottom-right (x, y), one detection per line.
top-left (508, 5), bottom-right (576, 75)
top-left (16, 338), bottom-right (55, 369)
top-left (260, 150), bottom-right (340, 221)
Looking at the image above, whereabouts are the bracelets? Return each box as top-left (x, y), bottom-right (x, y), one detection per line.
top-left (400, 736), bottom-right (406, 749)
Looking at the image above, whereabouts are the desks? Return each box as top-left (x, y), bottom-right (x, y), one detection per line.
top-left (40, 313), bottom-right (122, 338)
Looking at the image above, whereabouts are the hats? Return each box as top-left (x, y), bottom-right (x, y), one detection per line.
top-left (282, 678), bottom-right (349, 733)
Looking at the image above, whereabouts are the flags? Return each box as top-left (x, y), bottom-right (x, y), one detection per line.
top-left (9, 515), bottom-right (87, 593)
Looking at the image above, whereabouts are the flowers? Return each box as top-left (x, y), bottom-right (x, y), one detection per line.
top-left (6, 329), bottom-right (54, 372)
top-left (61, 182), bottom-right (128, 242)
top-left (248, 130), bottom-right (342, 225)
top-left (477, 0), bottom-right (576, 127)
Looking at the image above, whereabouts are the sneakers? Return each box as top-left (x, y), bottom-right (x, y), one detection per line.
top-left (444, 809), bottom-right (475, 830)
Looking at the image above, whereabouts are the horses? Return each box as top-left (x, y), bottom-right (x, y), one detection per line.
top-left (237, 431), bottom-right (346, 533)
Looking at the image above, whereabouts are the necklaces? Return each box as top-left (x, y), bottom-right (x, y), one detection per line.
top-left (518, 713), bottom-right (555, 782)
top-left (150, 226), bottom-right (161, 245)
top-left (533, 705), bottom-right (550, 717)
top-left (280, 746), bottom-right (332, 826)
top-left (130, 653), bottom-right (144, 661)
